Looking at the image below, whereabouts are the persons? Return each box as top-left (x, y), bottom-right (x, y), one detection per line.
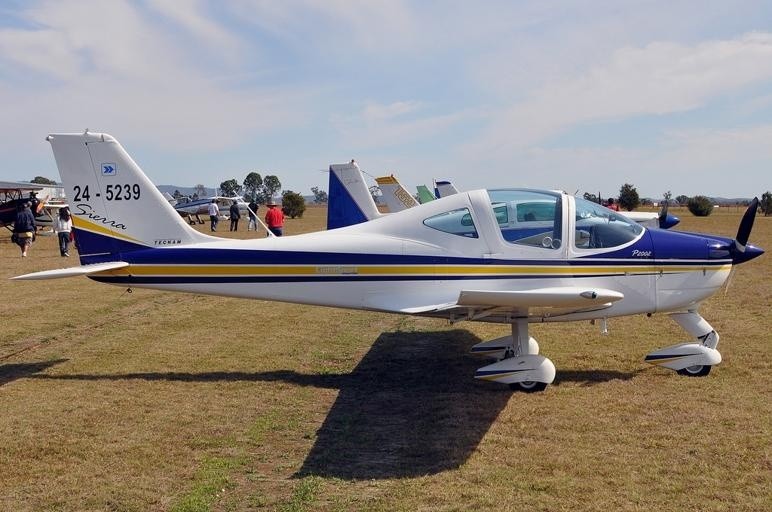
top-left (53, 207), bottom-right (72, 256)
top-left (230, 199), bottom-right (241, 230)
top-left (248, 198), bottom-right (260, 230)
top-left (606, 198), bottom-right (618, 208)
top-left (14, 202), bottom-right (36, 257)
top-left (208, 198), bottom-right (220, 231)
top-left (265, 201), bottom-right (285, 234)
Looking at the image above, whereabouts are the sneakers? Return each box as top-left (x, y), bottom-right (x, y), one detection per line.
top-left (61, 252), bottom-right (69, 257)
top-left (22, 252), bottom-right (26, 257)
top-left (211, 228), bottom-right (216, 232)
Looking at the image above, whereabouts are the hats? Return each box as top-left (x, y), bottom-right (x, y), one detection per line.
top-left (250, 199), bottom-right (256, 202)
top-left (266, 200), bottom-right (277, 208)
top-left (233, 200), bottom-right (238, 204)
